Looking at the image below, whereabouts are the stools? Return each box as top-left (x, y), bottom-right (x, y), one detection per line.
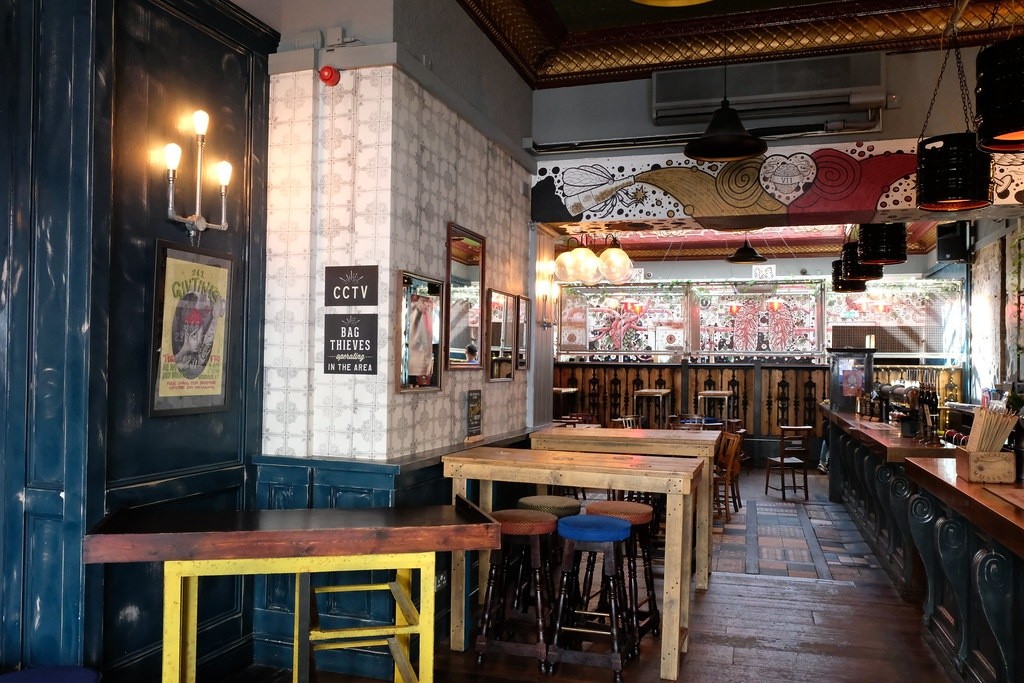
top-left (474, 495), bottom-right (659, 683)
top-left (697, 390), bottom-right (732, 433)
top-left (633, 388), bottom-right (672, 430)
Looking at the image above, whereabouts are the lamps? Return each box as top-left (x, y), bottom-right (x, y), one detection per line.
top-left (975, 0), bottom-right (1024, 154)
top-left (841, 229), bottom-right (884, 280)
top-left (553, 229), bottom-right (634, 286)
top-left (725, 228), bottom-right (768, 266)
top-left (680, 12), bottom-right (770, 165)
top-left (831, 258), bottom-right (868, 294)
top-left (164, 109), bottom-right (236, 234)
top-left (541, 281), bottom-right (559, 331)
top-left (914, 22), bottom-right (996, 213)
top-left (856, 222), bottom-right (908, 266)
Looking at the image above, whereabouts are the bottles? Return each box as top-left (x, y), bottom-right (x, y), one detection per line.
top-left (855, 381), bottom-right (945, 447)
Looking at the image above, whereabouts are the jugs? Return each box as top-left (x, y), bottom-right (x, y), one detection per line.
top-left (989, 390), bottom-right (1004, 400)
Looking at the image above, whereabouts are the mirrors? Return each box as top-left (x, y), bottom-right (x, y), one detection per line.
top-left (395, 268), bottom-right (443, 394)
top-left (517, 293), bottom-right (531, 372)
top-left (484, 285), bottom-right (516, 383)
top-left (444, 219), bottom-right (487, 371)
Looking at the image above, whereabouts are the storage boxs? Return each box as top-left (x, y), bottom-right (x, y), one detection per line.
top-left (955, 446), bottom-right (1017, 484)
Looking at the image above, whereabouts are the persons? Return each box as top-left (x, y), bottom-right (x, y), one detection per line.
top-left (817, 440), bottom-right (830, 474)
top-left (465, 344), bottom-right (479, 364)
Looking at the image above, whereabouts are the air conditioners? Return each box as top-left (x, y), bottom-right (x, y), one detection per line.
top-left (652, 47), bottom-right (888, 127)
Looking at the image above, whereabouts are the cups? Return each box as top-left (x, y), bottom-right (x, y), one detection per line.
top-left (901, 416), bottom-right (919, 438)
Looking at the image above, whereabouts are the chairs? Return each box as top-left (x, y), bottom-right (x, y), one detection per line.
top-left (613, 415), bottom-right (815, 521)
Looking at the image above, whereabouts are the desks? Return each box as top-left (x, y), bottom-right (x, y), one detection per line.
top-left (81, 491), bottom-right (504, 683)
top-left (553, 388), bottom-right (579, 417)
top-left (441, 444), bottom-right (705, 680)
top-left (527, 429), bottom-right (723, 590)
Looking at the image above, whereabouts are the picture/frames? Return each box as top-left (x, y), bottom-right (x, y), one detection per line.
top-left (145, 236), bottom-right (234, 420)
